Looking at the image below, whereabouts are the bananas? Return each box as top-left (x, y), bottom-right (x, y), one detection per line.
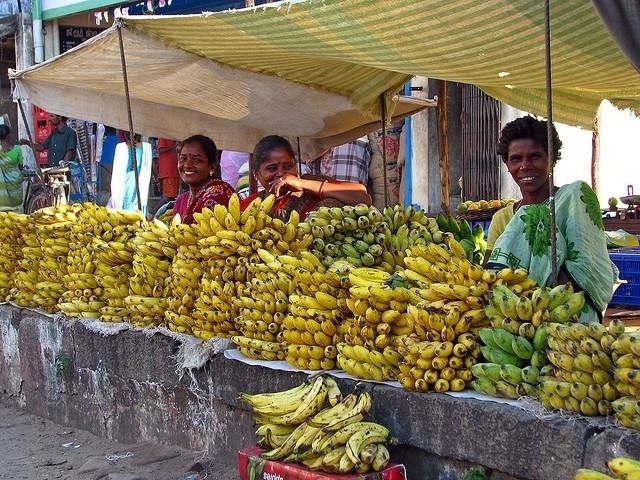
top-left (574, 457), bottom-right (640, 478)
top-left (0, 193), bottom-right (640, 429)
top-left (237, 371), bottom-right (390, 473)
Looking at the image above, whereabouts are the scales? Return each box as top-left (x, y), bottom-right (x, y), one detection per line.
top-left (619, 194), bottom-right (640, 219)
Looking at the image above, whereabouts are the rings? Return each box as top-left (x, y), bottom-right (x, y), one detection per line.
top-left (279, 178), bottom-right (282, 182)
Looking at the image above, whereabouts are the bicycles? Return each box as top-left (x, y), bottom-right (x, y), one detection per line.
top-left (21, 164), bottom-right (53, 215)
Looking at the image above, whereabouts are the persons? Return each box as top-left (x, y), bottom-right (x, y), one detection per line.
top-left (240, 133), bottom-right (372, 224)
top-left (482, 112), bottom-right (621, 325)
top-left (157, 137), bottom-right (181, 202)
top-left (16, 115), bottom-right (77, 198)
top-left (173, 135), bottom-right (240, 226)
top-left (107, 130), bottom-right (153, 218)
top-left (0, 124), bottom-right (25, 212)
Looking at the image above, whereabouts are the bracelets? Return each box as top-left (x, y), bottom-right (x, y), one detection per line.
top-left (319, 180), bottom-right (329, 196)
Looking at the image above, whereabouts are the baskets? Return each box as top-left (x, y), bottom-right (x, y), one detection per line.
top-left (608, 249), bottom-right (640, 307)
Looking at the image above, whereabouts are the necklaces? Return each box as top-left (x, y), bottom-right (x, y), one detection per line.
top-left (186, 194), bottom-right (195, 207)
top-left (513, 191), bottom-right (551, 215)
